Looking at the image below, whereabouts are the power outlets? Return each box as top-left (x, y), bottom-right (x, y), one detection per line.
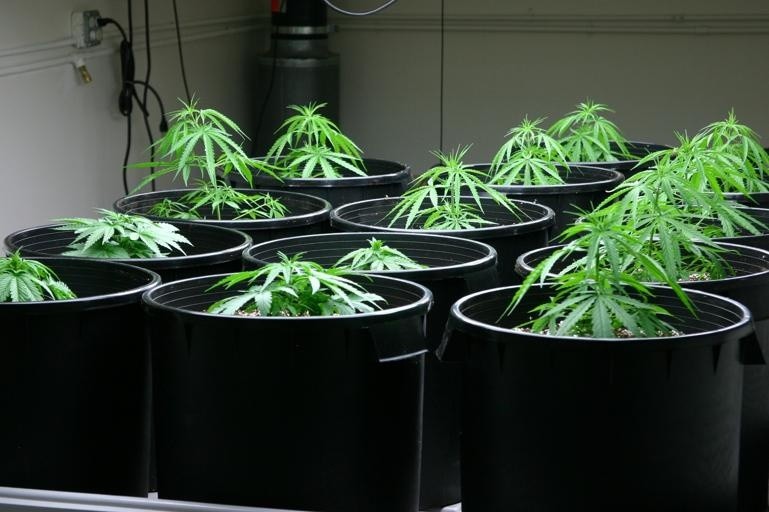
top-left (71, 9), bottom-right (103, 48)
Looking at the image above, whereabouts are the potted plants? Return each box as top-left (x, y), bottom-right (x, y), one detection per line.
top-left (113, 91), bottom-right (334, 247)
top-left (432, 202), bottom-right (763, 511)
top-left (330, 142), bottom-right (557, 292)
top-left (0, 250), bottom-right (162, 498)
top-left (650, 105), bottom-right (769, 208)
top-left (575, 129), bottom-right (768, 250)
top-left (143, 251), bottom-right (434, 512)
top-left (0, 206), bottom-right (252, 492)
top-left (239, 231), bottom-right (499, 506)
top-left (427, 113), bottom-right (625, 250)
top-left (513, 151), bottom-right (769, 510)
top-left (214, 102), bottom-right (414, 231)
top-left (507, 97), bottom-right (679, 199)
